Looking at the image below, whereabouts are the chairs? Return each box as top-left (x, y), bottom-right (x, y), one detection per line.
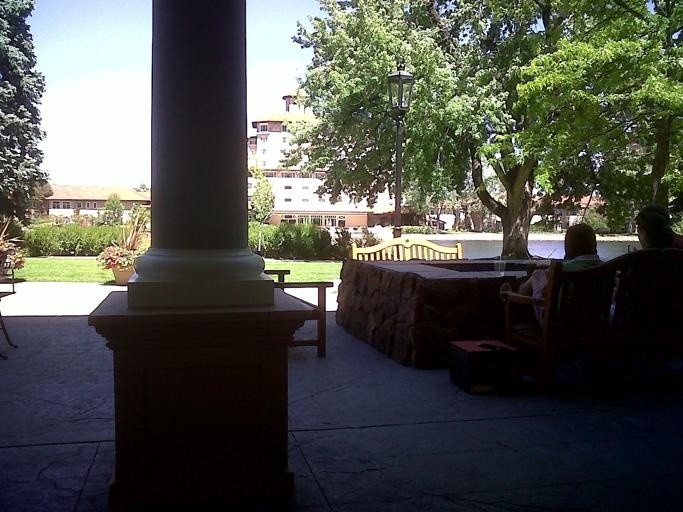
top-left (1, 263), bottom-right (18, 360)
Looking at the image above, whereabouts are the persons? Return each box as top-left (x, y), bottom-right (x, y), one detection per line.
top-left (606, 202), bottom-right (682, 330)
top-left (498, 223), bottom-right (602, 337)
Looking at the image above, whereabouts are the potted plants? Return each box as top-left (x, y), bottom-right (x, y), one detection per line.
top-left (0, 215), bottom-right (26, 276)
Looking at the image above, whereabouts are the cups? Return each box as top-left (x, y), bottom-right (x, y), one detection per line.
top-left (494, 261), bottom-right (506, 277)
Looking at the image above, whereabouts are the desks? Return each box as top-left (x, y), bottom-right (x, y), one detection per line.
top-left (448, 339), bottom-right (527, 394)
top-left (337, 258), bottom-right (566, 369)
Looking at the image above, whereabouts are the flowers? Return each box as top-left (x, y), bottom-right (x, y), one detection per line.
top-left (96, 208), bottom-right (149, 270)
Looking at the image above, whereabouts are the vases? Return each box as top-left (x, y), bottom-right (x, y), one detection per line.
top-left (112, 265), bottom-right (134, 285)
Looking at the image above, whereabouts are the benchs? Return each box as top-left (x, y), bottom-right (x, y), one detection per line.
top-left (499, 248), bottom-right (683, 393)
top-left (264, 270), bottom-right (333, 357)
top-left (351, 237), bottom-right (463, 261)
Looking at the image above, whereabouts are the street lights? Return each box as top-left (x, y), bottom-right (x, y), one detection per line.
top-left (386, 56), bottom-right (413, 258)
